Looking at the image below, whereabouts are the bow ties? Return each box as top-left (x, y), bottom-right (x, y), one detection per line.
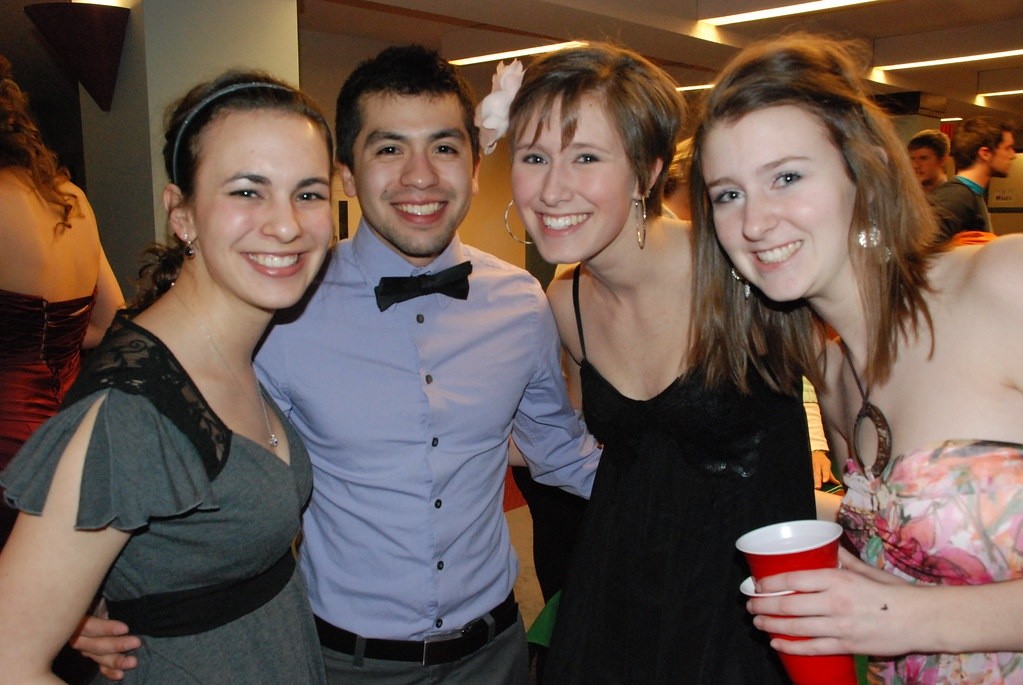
top-left (373, 261), bottom-right (473, 312)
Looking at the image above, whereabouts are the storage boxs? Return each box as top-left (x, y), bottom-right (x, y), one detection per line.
top-left (988, 153), bottom-right (1023, 237)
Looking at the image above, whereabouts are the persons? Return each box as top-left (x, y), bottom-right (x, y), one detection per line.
top-left (680, 35), bottom-right (1023, 685)
top-left (1, 70), bottom-right (341, 685)
top-left (927, 116), bottom-right (1016, 251)
top-left (2, 84), bottom-right (131, 475)
top-left (505, 40), bottom-right (851, 685)
top-left (906, 129), bottom-right (950, 195)
top-left (67, 46), bottom-right (602, 685)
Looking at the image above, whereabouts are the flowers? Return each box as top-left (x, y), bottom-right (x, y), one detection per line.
top-left (474, 58), bottom-right (528, 155)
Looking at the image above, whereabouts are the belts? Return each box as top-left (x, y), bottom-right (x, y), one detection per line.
top-left (312, 586), bottom-right (520, 664)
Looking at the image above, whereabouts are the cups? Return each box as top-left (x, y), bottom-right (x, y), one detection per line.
top-left (736, 520), bottom-right (843, 593)
top-left (740, 577), bottom-right (858, 685)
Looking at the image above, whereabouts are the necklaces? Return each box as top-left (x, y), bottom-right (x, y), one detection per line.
top-left (838, 338), bottom-right (892, 477)
top-left (169, 282), bottom-right (280, 445)
